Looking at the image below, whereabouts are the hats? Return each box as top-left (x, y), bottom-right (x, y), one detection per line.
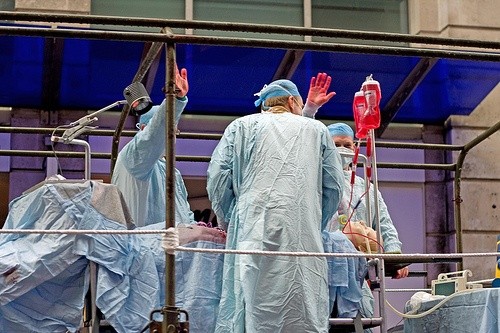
top-left (327, 123), bottom-right (355, 140)
top-left (136, 105), bottom-right (160, 129)
top-left (254, 80), bottom-right (300, 103)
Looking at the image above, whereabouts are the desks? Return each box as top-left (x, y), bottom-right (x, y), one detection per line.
top-left (403, 287), bottom-right (500, 333)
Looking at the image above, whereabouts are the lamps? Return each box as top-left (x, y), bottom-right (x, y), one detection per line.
top-left (60, 82), bottom-right (153, 141)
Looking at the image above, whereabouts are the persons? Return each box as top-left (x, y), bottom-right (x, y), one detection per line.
top-left (207, 80), bottom-right (345, 333)
top-left (111, 68), bottom-right (195, 227)
top-left (303, 73), bottom-right (409, 318)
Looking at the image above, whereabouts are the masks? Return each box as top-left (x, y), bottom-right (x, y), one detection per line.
top-left (336, 146), bottom-right (355, 169)
